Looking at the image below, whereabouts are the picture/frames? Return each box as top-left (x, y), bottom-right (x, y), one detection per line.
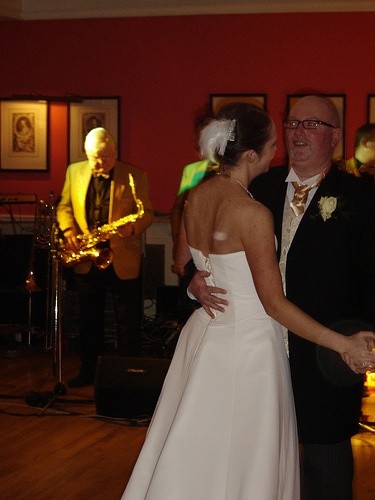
top-left (0, 96), bottom-right (50, 173)
top-left (210, 94), bottom-right (266, 119)
top-left (286, 94), bottom-right (346, 163)
top-left (67, 96), bottom-right (121, 165)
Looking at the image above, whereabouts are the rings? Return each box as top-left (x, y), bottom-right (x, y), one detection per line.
top-left (360, 362), bottom-right (367, 369)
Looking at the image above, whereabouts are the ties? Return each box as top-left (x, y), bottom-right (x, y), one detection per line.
top-left (290, 180), bottom-right (320, 215)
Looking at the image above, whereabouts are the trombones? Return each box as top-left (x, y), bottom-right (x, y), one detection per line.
top-left (17, 190), bottom-right (58, 355)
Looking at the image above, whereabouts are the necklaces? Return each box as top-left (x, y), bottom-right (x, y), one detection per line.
top-left (216, 170), bottom-right (256, 201)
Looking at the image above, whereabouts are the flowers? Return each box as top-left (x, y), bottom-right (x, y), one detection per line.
top-left (317, 194), bottom-right (338, 222)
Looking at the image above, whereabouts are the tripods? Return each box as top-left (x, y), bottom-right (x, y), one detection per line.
top-left (0, 254), bottom-right (96, 417)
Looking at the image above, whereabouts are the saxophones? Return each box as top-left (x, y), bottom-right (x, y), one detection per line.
top-left (60, 173), bottom-right (144, 270)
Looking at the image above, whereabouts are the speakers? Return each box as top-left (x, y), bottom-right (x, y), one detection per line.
top-left (94, 357), bottom-right (172, 420)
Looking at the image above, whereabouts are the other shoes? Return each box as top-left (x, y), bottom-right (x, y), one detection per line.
top-left (68, 369), bottom-right (96, 389)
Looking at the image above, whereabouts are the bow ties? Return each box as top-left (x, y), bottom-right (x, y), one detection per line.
top-left (93, 170), bottom-right (110, 179)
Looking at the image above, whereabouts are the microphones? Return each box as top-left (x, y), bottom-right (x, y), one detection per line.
top-left (53, 222), bottom-right (59, 258)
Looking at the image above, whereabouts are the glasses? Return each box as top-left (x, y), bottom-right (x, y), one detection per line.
top-left (282, 118), bottom-right (336, 129)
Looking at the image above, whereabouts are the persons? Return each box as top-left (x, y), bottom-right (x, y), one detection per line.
top-left (336, 122), bottom-right (375, 178)
top-left (56, 127), bottom-right (153, 389)
top-left (121, 102), bottom-right (375, 500)
top-left (172, 117), bottom-right (219, 326)
top-left (182, 94), bottom-right (375, 500)
